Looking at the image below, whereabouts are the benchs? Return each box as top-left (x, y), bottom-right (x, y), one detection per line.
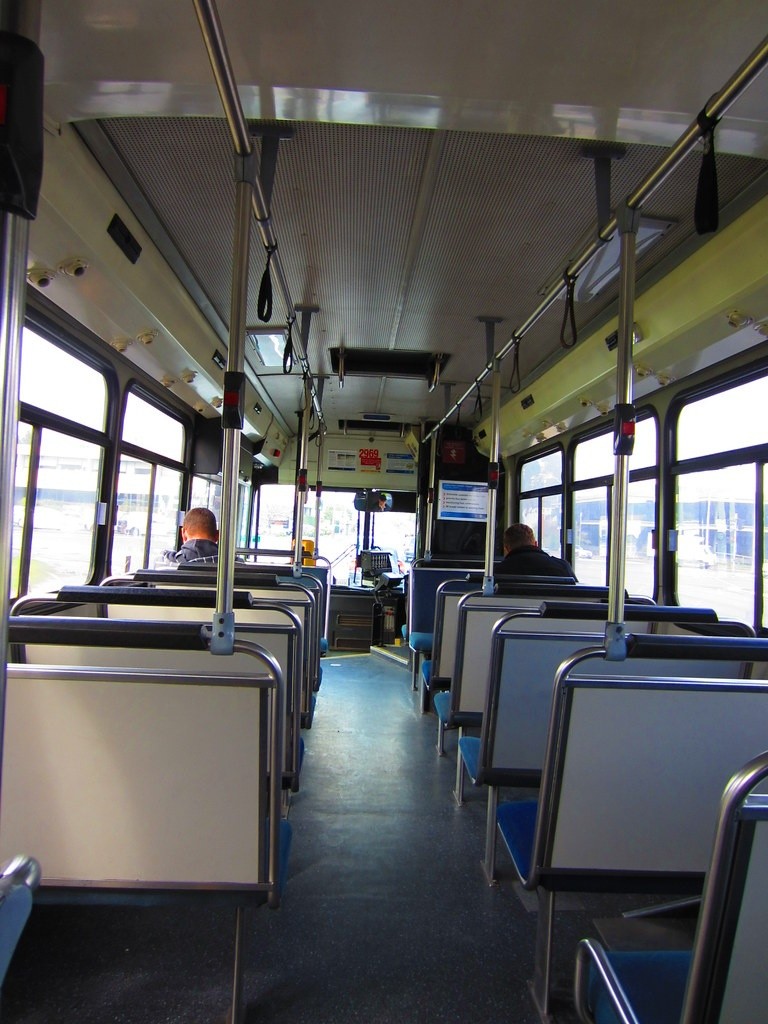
top-left (1, 554), bottom-right (768, 1023)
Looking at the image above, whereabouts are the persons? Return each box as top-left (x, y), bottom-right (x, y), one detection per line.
top-left (493, 523), bottom-right (578, 585)
top-left (153, 508), bottom-right (245, 571)
top-left (369, 495), bottom-right (391, 512)
